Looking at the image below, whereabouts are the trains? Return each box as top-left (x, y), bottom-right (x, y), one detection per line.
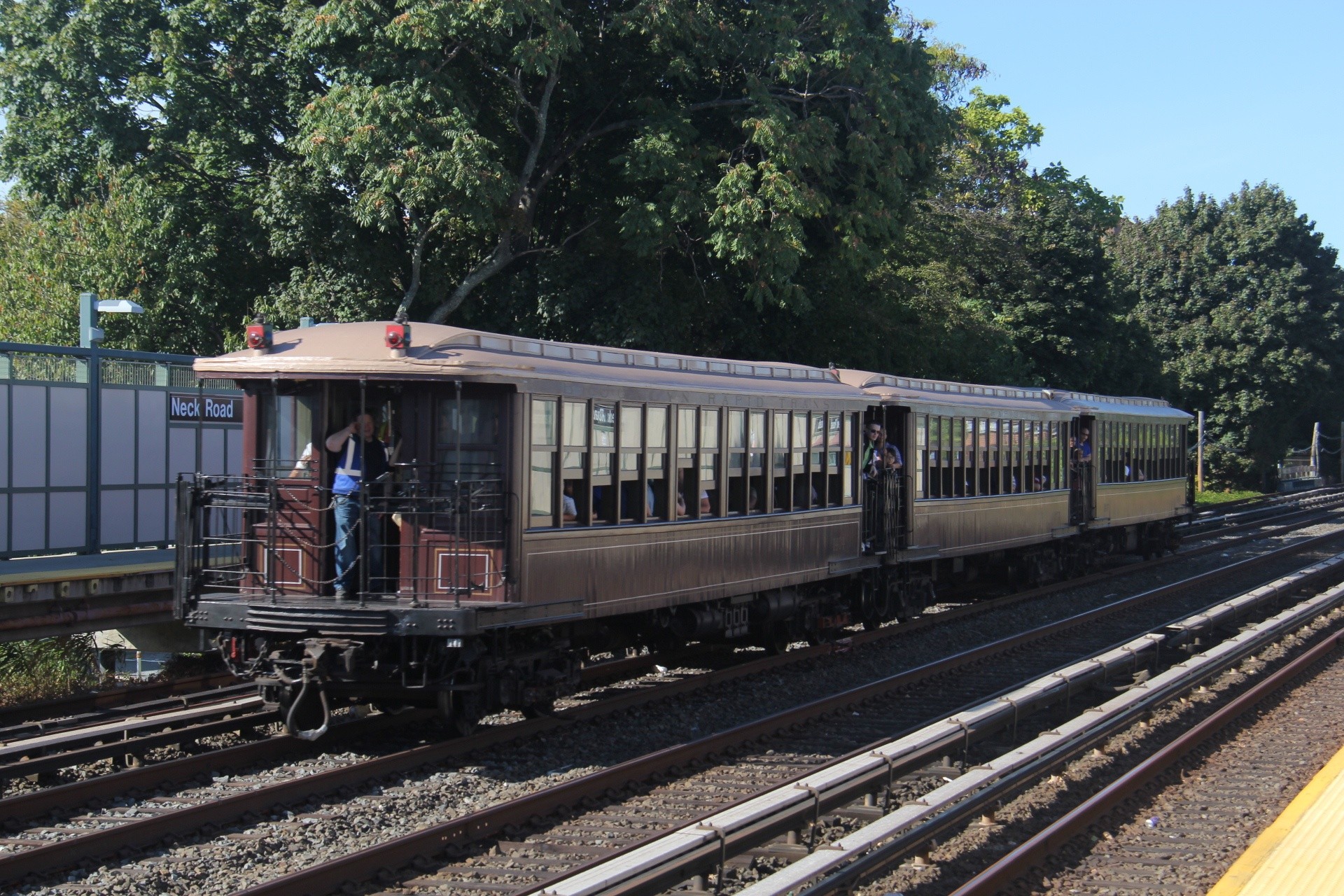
top-left (163, 305), bottom-right (1198, 745)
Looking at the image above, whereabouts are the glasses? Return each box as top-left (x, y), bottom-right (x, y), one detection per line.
top-left (868, 429), bottom-right (880, 434)
top-left (1081, 432), bottom-right (1088, 436)
top-left (886, 455), bottom-right (895, 460)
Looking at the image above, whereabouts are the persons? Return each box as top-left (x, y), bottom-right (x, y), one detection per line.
top-left (289, 410), bottom-right (1184, 602)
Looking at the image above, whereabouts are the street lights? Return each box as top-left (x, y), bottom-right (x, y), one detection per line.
top-left (79, 291), bottom-right (144, 350)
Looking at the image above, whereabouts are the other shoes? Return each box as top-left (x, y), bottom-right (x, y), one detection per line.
top-left (336, 588), bottom-right (348, 598)
